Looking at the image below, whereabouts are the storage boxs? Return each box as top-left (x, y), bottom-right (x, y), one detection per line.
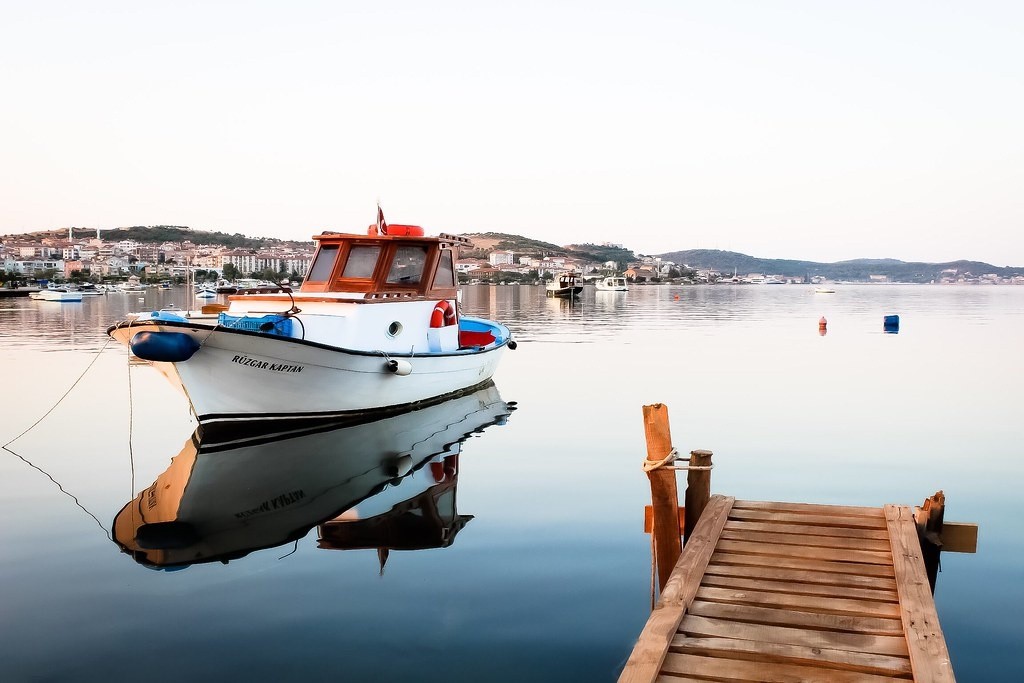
top-left (217, 312), bottom-right (293, 339)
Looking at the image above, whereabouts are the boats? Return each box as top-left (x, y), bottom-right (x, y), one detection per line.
top-left (545, 272), bottom-right (585, 299)
top-left (80, 204), bottom-right (520, 441)
top-left (111, 425), bottom-right (522, 574)
top-left (596, 276), bottom-right (631, 291)
top-left (28, 285), bottom-right (83, 302)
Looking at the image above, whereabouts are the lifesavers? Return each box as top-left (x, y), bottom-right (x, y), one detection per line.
top-left (431, 453), bottom-right (460, 484)
top-left (429, 301), bottom-right (459, 328)
